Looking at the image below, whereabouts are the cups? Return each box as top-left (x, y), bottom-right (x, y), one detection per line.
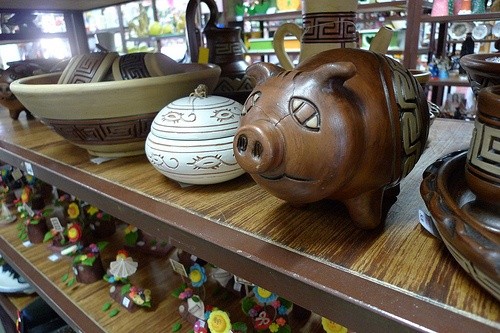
top-left (465, 85), bottom-right (500, 206)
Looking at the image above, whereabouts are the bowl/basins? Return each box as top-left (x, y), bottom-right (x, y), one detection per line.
top-left (9, 51), bottom-right (222, 159)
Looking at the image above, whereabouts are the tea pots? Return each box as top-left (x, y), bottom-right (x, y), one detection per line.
top-left (272, 0), bottom-right (394, 72)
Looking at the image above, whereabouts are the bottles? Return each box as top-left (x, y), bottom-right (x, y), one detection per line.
top-left (144, 83), bottom-right (249, 184)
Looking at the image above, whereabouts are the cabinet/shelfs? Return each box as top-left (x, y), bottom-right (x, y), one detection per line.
top-left (0, 1), bottom-right (500, 333)
